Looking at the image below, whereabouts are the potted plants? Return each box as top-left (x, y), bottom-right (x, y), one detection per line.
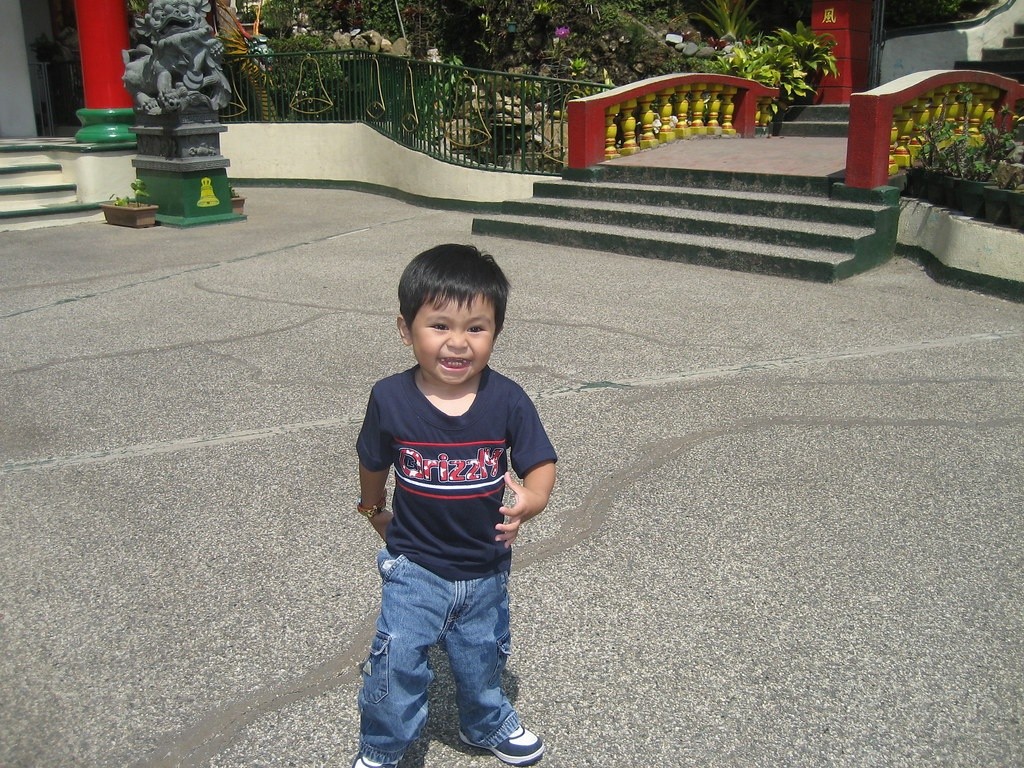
top-left (99, 179), bottom-right (161, 228)
top-left (227, 183), bottom-right (247, 215)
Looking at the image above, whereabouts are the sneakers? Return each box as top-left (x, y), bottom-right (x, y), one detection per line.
top-left (459, 723), bottom-right (544, 766)
top-left (352, 752), bottom-right (405, 768)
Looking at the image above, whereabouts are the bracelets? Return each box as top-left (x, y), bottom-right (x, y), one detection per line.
top-left (358, 487), bottom-right (386, 519)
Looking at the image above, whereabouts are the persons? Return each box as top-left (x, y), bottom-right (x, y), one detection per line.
top-left (349, 242), bottom-right (557, 768)
